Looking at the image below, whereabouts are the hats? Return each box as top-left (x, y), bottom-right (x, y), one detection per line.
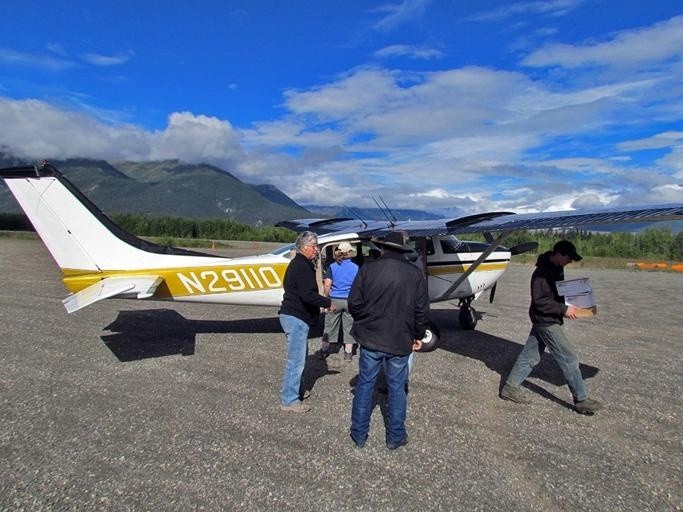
top-left (554, 240), bottom-right (582, 261)
top-left (338, 242), bottom-right (358, 257)
top-left (370, 232), bottom-right (413, 253)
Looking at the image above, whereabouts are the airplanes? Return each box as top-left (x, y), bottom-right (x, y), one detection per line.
top-left (0, 159), bottom-right (683, 352)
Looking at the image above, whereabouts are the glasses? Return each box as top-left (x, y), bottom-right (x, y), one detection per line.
top-left (306, 244), bottom-right (319, 248)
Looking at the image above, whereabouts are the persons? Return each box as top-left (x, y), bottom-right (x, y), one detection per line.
top-left (318, 242), bottom-right (361, 363)
top-left (500, 240), bottom-right (603, 413)
top-left (278, 231), bottom-right (337, 413)
top-left (347, 232), bottom-right (429, 449)
top-left (377, 229), bottom-right (424, 396)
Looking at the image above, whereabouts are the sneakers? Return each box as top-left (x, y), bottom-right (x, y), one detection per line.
top-left (318, 348), bottom-right (330, 359)
top-left (281, 390), bottom-right (310, 413)
top-left (344, 352), bottom-right (352, 360)
top-left (500, 383), bottom-right (529, 404)
top-left (574, 398), bottom-right (602, 414)
